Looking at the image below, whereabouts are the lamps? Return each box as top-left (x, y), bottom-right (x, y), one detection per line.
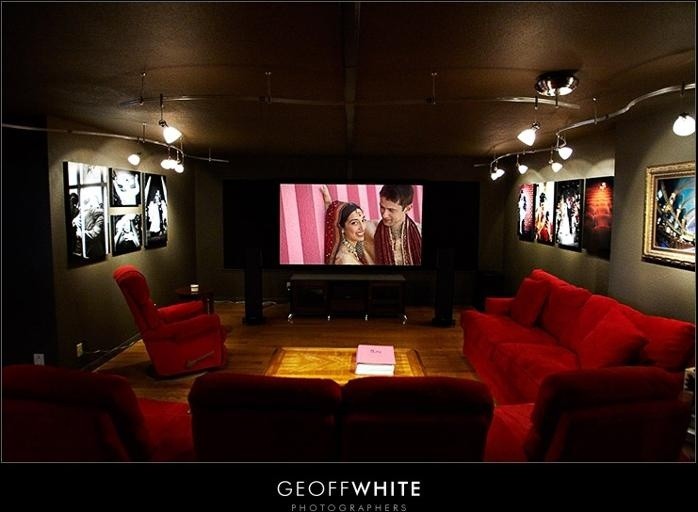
top-left (488, 70), bottom-right (581, 182)
top-left (671, 84), bottom-right (696, 137)
top-left (127, 93), bottom-right (186, 174)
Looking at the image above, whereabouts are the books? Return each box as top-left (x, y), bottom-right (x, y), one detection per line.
top-left (355, 344), bottom-right (396, 376)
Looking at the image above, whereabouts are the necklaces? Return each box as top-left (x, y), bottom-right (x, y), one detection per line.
top-left (341, 240), bottom-right (360, 261)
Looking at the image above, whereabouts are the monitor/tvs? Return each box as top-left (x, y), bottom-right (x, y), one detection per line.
top-left (276, 178), bottom-right (427, 273)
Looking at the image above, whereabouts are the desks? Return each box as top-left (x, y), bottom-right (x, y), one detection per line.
top-left (174, 285), bottom-right (214, 315)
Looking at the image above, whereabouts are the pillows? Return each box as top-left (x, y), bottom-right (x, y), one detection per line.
top-left (510, 277), bottom-right (553, 327)
top-left (579, 304), bottom-right (649, 369)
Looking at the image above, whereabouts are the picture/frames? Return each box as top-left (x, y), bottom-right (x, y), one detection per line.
top-left (640, 160), bottom-right (696, 272)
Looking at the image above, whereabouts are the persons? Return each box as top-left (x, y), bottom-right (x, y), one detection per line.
top-left (535, 193), bottom-right (552, 242)
top-left (518, 190), bottom-right (526, 235)
top-left (324, 200), bottom-right (374, 266)
top-left (320, 184), bottom-right (422, 266)
top-left (557, 195), bottom-right (578, 245)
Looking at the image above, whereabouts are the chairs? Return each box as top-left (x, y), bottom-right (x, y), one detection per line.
top-left (343, 376), bottom-right (494, 462)
top-left (111, 265), bottom-right (228, 378)
top-left (2, 362), bottom-right (194, 460)
top-left (483, 365), bottom-right (692, 462)
top-left (187, 370), bottom-right (341, 462)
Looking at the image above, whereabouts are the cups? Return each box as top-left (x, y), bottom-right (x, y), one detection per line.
top-left (190, 285), bottom-right (199, 293)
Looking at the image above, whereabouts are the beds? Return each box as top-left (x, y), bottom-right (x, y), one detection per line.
top-left (265, 346), bottom-right (427, 387)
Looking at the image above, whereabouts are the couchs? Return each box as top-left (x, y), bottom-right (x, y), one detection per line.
top-left (459, 268), bottom-right (695, 405)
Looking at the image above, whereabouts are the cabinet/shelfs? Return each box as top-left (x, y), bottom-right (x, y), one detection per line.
top-left (288, 268), bottom-right (406, 317)
